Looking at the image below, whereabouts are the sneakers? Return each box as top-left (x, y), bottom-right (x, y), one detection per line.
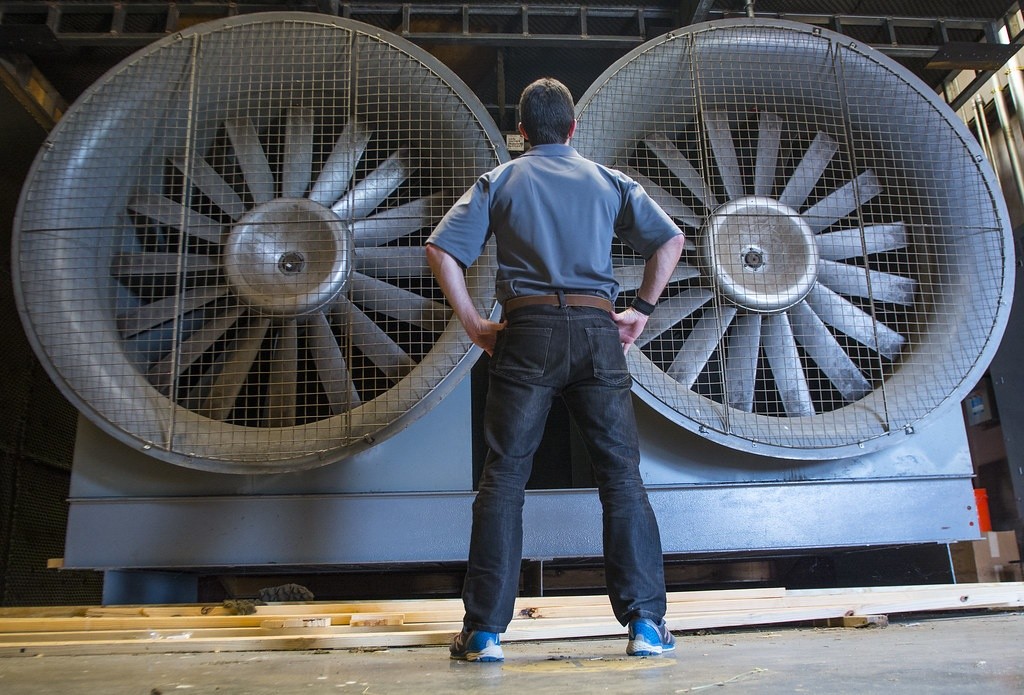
top-left (626, 617), bottom-right (675, 655)
top-left (449, 626), bottom-right (504, 662)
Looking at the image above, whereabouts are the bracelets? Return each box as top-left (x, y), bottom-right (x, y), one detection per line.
top-left (630, 296), bottom-right (656, 316)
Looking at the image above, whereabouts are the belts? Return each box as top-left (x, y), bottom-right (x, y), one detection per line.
top-left (501, 294), bottom-right (612, 315)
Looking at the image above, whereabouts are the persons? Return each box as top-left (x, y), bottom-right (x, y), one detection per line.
top-left (416, 75), bottom-right (688, 661)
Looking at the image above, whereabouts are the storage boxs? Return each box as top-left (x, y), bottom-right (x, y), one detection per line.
top-left (973, 488), bottom-right (992, 532)
top-left (964, 378), bottom-right (999, 427)
top-left (949, 530), bottom-right (1020, 584)
